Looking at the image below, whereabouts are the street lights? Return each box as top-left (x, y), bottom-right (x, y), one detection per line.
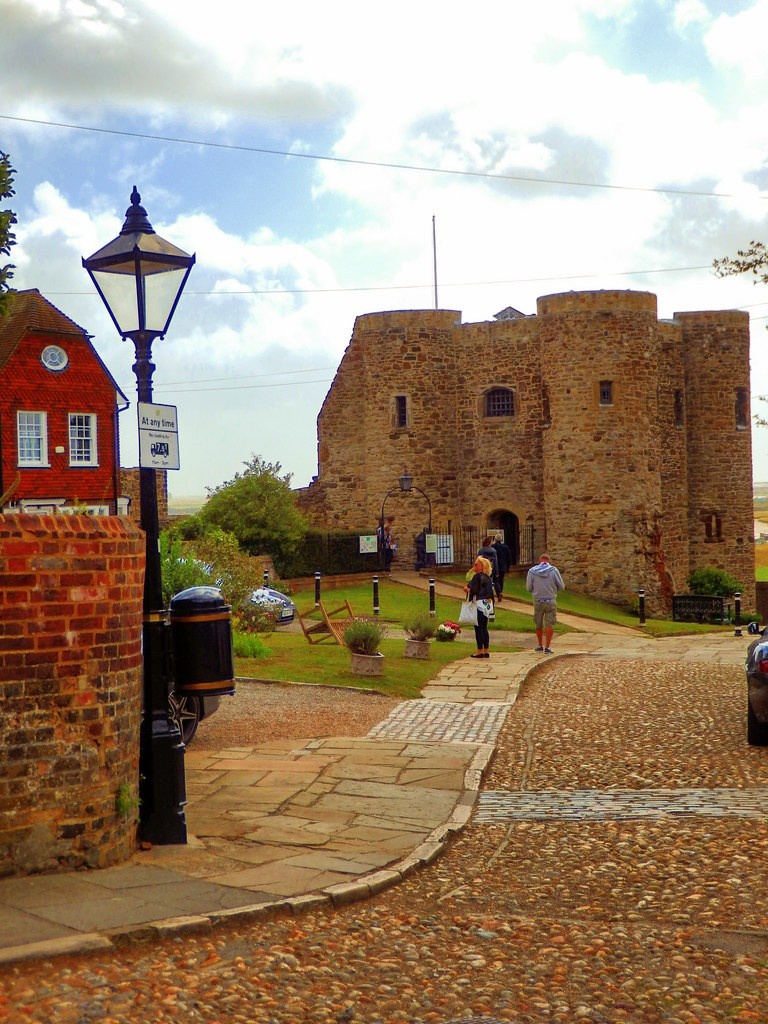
top-left (78, 186), bottom-right (240, 852)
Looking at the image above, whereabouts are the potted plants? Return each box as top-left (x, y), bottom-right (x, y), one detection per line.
top-left (401, 609), bottom-right (440, 659)
top-left (341, 613), bottom-right (387, 675)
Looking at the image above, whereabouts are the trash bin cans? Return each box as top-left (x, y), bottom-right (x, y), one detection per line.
top-left (169, 585), bottom-right (237, 699)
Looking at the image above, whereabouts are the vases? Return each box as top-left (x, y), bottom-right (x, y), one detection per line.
top-left (436, 635), bottom-right (456, 641)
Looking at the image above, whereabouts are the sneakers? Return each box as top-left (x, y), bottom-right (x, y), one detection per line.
top-left (545, 648), bottom-right (555, 654)
top-left (535, 646), bottom-right (543, 651)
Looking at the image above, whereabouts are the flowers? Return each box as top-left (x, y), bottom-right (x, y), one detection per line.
top-left (431, 621), bottom-right (461, 639)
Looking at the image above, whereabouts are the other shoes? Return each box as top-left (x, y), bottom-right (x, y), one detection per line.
top-left (483, 653), bottom-right (490, 658)
top-left (470, 653), bottom-right (484, 658)
top-left (498, 593), bottom-right (503, 602)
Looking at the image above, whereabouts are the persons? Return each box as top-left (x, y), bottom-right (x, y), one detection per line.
top-left (525, 554), bottom-right (565, 654)
top-left (379, 524), bottom-right (395, 572)
top-left (476, 537), bottom-right (499, 605)
top-left (463, 556), bottom-right (494, 658)
top-left (489, 534), bottom-right (511, 602)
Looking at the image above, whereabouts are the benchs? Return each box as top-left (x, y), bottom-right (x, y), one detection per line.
top-left (295, 598), bottom-right (355, 647)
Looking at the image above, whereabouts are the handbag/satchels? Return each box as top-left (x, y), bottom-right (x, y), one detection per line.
top-left (458, 588), bottom-right (478, 626)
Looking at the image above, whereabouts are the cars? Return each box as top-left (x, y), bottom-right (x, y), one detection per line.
top-left (745, 621), bottom-right (768, 747)
